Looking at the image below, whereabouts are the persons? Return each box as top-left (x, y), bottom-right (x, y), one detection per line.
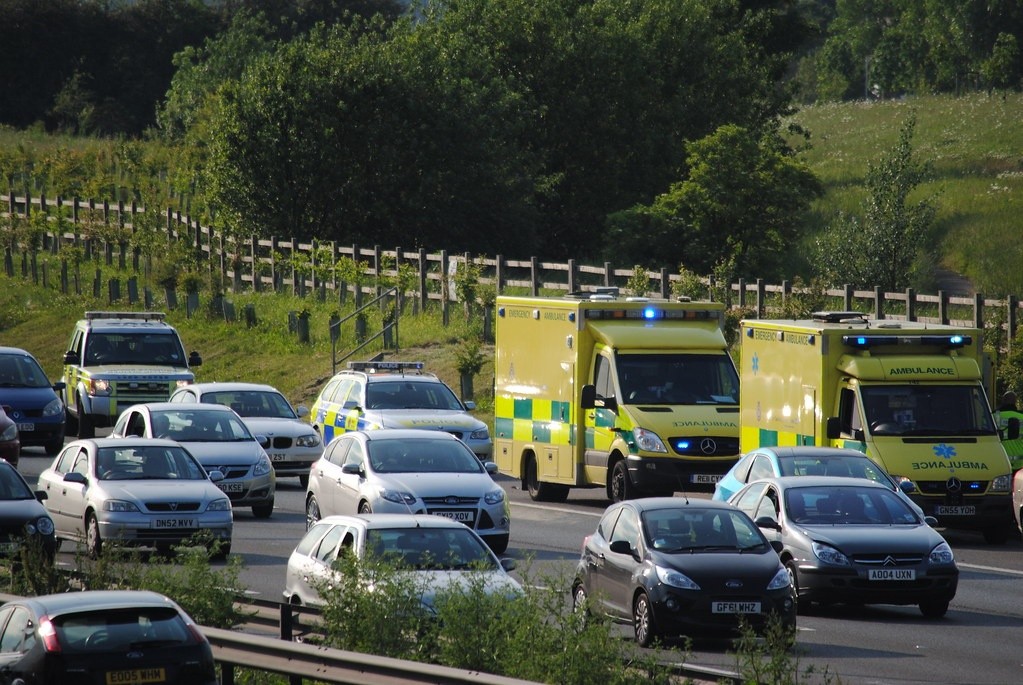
top-left (992, 391), bottom-right (1023, 492)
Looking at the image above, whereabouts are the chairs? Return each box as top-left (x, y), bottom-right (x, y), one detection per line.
top-left (791, 495), bottom-right (806, 517)
top-left (855, 497), bottom-right (865, 516)
top-left (424, 540), bottom-right (450, 558)
top-left (368, 538), bottom-right (384, 559)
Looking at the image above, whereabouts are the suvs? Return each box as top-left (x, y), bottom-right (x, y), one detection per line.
top-left (59, 311), bottom-right (202, 439)
top-left (311, 370), bottom-right (493, 467)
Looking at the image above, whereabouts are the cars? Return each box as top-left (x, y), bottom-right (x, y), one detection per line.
top-left (0, 590), bottom-right (217, 685)
top-left (1013, 468), bottom-right (1023, 536)
top-left (305, 430), bottom-right (511, 554)
top-left (0, 406), bottom-right (20, 469)
top-left (0, 458), bottom-right (57, 565)
top-left (0, 346), bottom-right (67, 456)
top-left (713, 476), bottom-right (961, 617)
top-left (37, 439), bottom-right (233, 562)
top-left (571, 497), bottom-right (797, 649)
top-left (163, 382), bottom-right (324, 488)
top-left (106, 402), bottom-right (276, 519)
top-left (282, 512), bottom-right (530, 628)
top-left (710, 446), bottom-right (926, 522)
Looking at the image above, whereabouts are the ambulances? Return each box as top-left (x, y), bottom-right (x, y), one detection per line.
top-left (740, 311), bottom-right (1013, 545)
top-left (492, 295), bottom-right (740, 502)
